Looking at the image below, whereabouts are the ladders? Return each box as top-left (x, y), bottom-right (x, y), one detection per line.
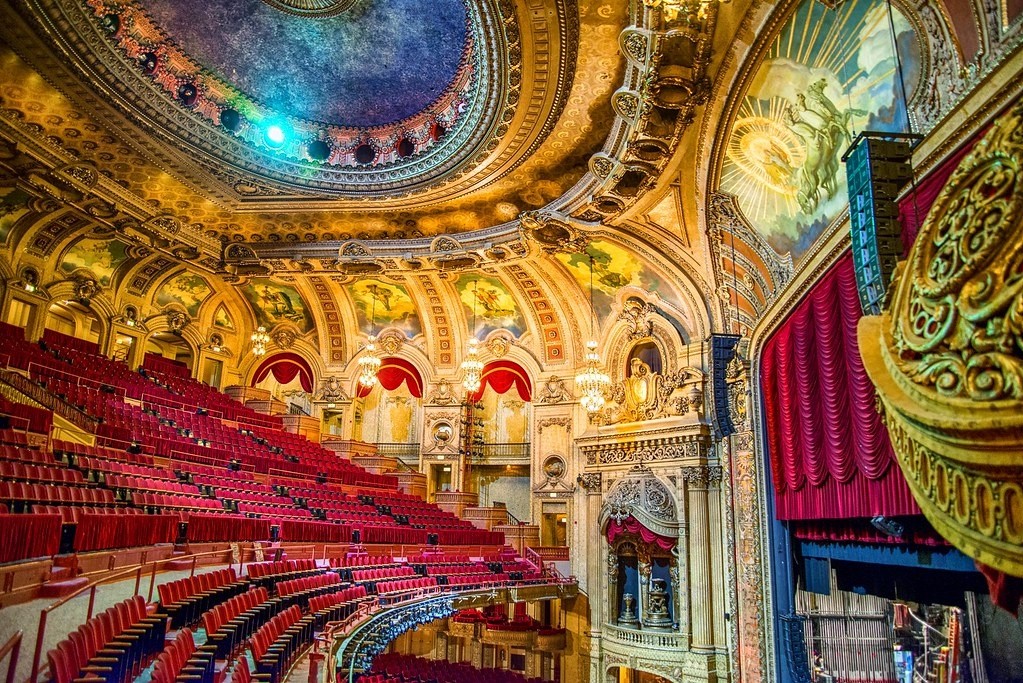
top-left (948, 614), bottom-right (958, 650)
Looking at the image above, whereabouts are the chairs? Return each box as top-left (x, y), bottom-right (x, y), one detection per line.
top-left (0, 310), bottom-right (578, 683)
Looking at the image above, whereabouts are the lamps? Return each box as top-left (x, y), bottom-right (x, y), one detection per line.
top-left (575, 254), bottom-right (610, 413)
top-left (358, 286), bottom-right (382, 386)
top-left (250, 281), bottom-right (271, 358)
top-left (458, 281), bottom-right (485, 392)
